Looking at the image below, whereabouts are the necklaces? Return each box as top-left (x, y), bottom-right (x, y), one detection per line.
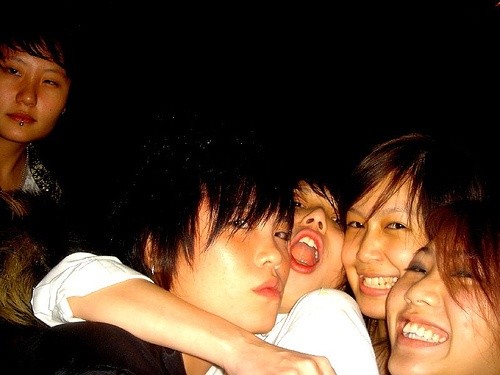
top-left (16, 147), bottom-right (30, 195)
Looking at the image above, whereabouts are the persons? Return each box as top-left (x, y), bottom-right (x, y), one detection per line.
top-left (0, 14), bottom-right (80, 206)
top-left (0, 132), bottom-right (500, 375)
